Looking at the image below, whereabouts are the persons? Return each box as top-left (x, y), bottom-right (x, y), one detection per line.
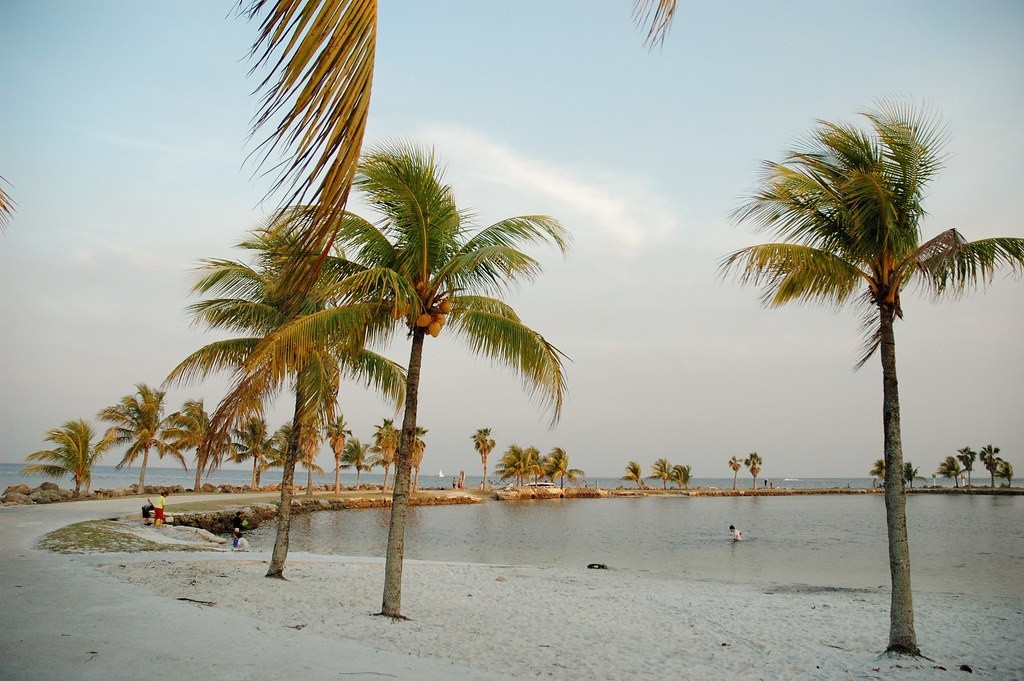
top-left (729, 526), bottom-right (742, 541)
top-left (873, 478), bottom-right (878, 488)
top-left (235, 531), bottom-right (249, 548)
top-left (233, 511), bottom-right (243, 547)
top-left (848, 484), bottom-right (850, 488)
top-left (142, 498), bottom-right (154, 526)
top-left (452, 477), bottom-right (462, 489)
top-left (154, 491), bottom-right (169, 525)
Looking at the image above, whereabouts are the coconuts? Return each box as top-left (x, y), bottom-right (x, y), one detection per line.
top-left (390, 282), bottom-right (452, 337)
